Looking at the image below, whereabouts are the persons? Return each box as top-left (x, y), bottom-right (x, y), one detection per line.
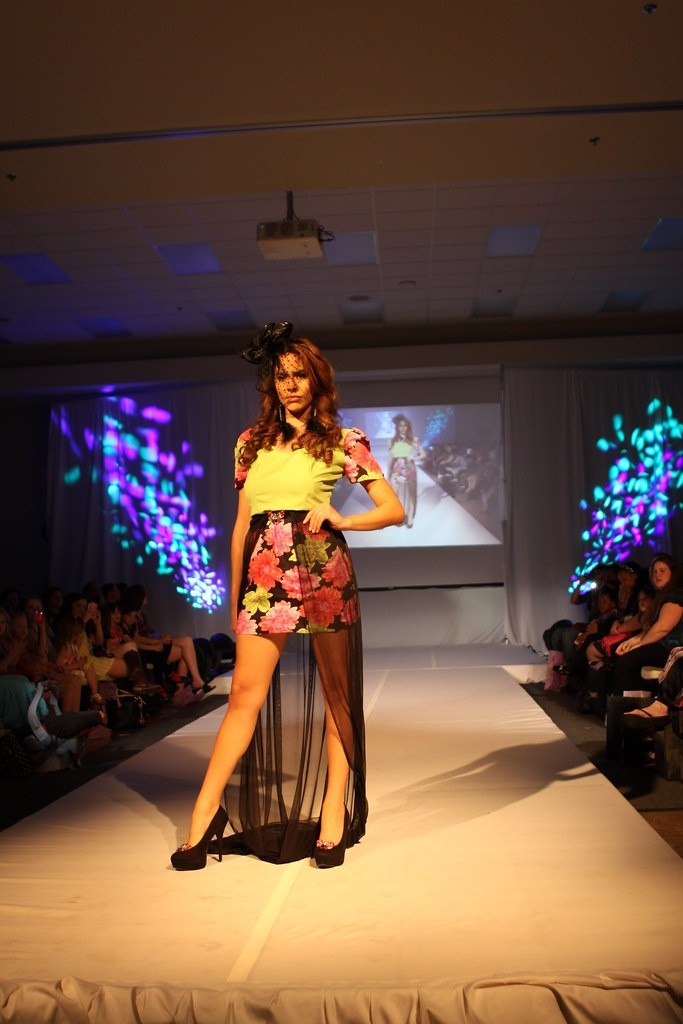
top-left (389, 413), bottom-right (427, 528)
top-left (0, 582), bottom-right (218, 786)
top-left (171, 323), bottom-right (406, 870)
top-left (553, 553), bottom-right (682, 729)
top-left (423, 442), bottom-right (500, 514)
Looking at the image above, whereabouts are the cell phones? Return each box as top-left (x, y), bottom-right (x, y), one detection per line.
top-left (88, 602), bottom-right (97, 618)
top-left (33, 608), bottom-right (44, 624)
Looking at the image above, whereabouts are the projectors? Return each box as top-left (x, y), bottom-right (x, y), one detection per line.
top-left (256, 218), bottom-right (325, 261)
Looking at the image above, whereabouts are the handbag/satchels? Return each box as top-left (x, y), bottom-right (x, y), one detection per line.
top-left (106, 689), bottom-right (146, 730)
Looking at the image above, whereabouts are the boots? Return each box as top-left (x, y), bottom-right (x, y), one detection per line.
top-left (123, 649), bottom-right (162, 693)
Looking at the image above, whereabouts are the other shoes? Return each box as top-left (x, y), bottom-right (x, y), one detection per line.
top-left (195, 682), bottom-right (216, 695)
top-left (171, 677), bottom-right (189, 684)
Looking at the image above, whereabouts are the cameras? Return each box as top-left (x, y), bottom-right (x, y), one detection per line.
top-left (584, 573), bottom-right (595, 580)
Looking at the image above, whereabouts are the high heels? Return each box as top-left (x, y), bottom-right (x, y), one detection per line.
top-left (173, 805), bottom-right (228, 870)
top-left (316, 806), bottom-right (349, 866)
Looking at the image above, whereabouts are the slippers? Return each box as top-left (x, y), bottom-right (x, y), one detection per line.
top-left (620, 707), bottom-right (674, 727)
